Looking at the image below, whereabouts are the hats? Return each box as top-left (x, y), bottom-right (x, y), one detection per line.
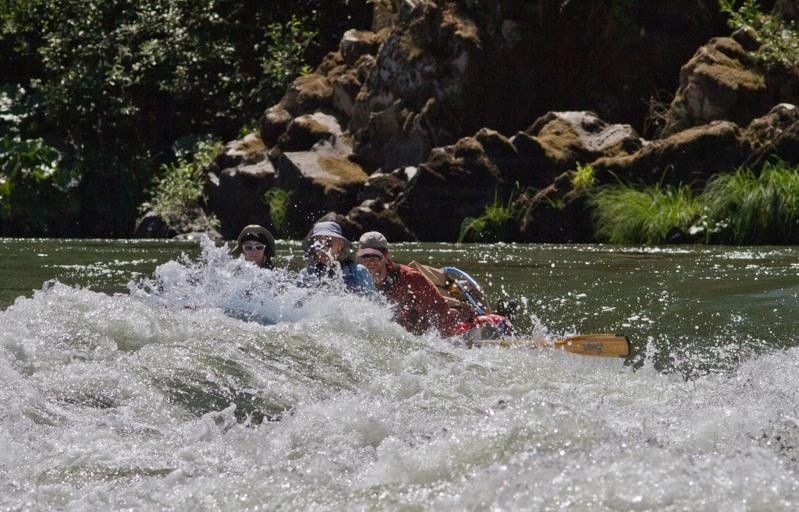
top-left (239, 231), bottom-right (268, 244)
top-left (357, 231), bottom-right (389, 258)
top-left (302, 220), bottom-right (352, 254)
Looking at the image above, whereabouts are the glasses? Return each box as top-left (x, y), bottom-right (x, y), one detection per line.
top-left (316, 239), bottom-right (334, 247)
top-left (362, 255), bottom-right (383, 261)
top-left (242, 244), bottom-right (267, 250)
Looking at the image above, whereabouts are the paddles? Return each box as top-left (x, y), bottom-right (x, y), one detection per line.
top-left (468, 334), bottom-right (632, 361)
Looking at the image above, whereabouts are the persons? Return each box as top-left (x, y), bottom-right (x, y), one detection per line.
top-left (299, 221), bottom-right (377, 300)
top-left (356, 230), bottom-right (449, 336)
top-left (228, 225), bottom-right (275, 270)
